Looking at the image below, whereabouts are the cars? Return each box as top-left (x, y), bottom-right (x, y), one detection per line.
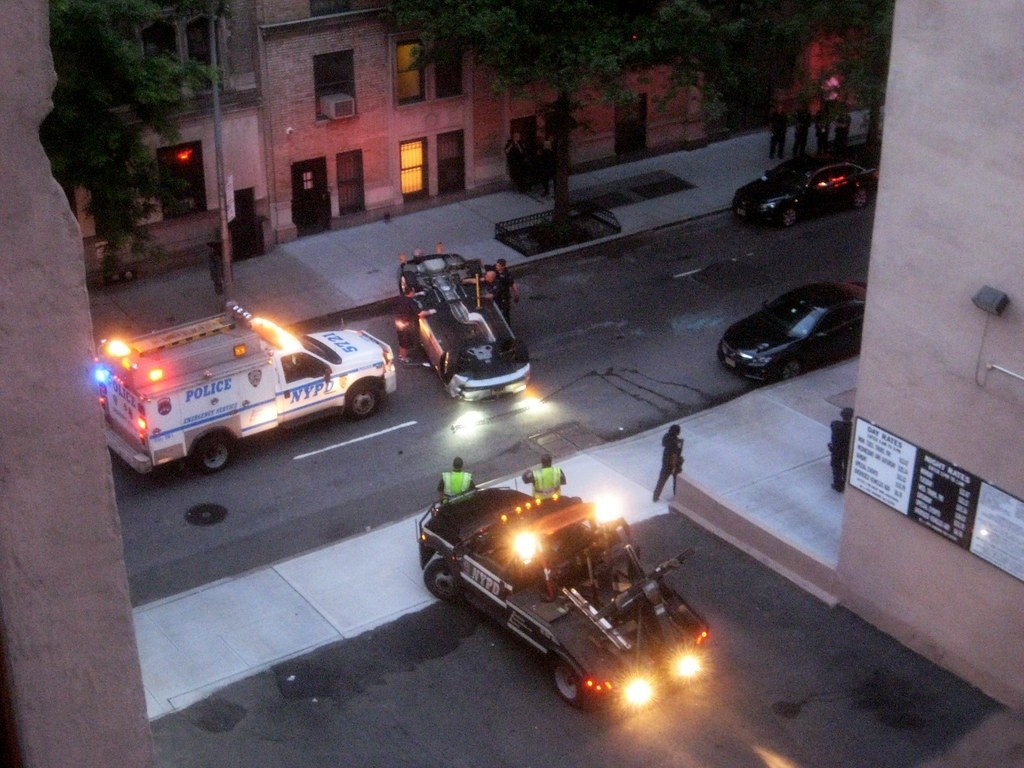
top-left (416, 484), bottom-right (713, 713)
top-left (730, 152), bottom-right (883, 226)
top-left (716, 278), bottom-right (868, 386)
top-left (393, 252), bottom-right (531, 403)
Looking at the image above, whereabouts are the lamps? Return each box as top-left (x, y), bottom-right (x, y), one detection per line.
top-left (973, 287), bottom-right (1008, 316)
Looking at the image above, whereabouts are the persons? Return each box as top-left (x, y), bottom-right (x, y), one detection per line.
top-left (834, 104), bottom-right (851, 139)
top-left (462, 259), bottom-right (520, 327)
top-left (814, 102), bottom-right (831, 155)
top-left (769, 105), bottom-right (788, 159)
top-left (522, 454), bottom-right (566, 502)
top-left (394, 286), bottom-right (437, 367)
top-left (504, 127), bottom-right (555, 198)
top-left (791, 100), bottom-right (812, 157)
top-left (437, 458), bottom-right (476, 498)
top-left (652, 425), bottom-right (685, 502)
top-left (827, 407), bottom-right (854, 493)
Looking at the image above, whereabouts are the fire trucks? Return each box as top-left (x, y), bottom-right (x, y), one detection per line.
top-left (91, 298), bottom-right (397, 478)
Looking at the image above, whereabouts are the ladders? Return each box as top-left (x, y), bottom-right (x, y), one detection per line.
top-left (130, 318), bottom-right (236, 355)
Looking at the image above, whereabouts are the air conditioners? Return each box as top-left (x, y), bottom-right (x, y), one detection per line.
top-left (320, 93), bottom-right (355, 119)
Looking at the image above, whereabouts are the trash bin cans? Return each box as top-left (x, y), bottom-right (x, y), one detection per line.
top-left (227, 212), bottom-right (270, 260)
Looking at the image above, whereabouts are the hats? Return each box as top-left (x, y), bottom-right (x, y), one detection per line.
top-left (453, 457), bottom-right (463, 468)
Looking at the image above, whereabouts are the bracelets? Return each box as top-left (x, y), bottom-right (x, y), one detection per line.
top-left (514, 295), bottom-right (519, 297)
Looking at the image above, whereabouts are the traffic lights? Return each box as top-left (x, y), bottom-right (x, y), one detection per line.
top-left (154, 138), bottom-right (208, 221)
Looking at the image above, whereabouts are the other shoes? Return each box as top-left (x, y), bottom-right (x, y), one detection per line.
top-left (398, 355), bottom-right (414, 362)
top-left (831, 484), bottom-right (841, 492)
